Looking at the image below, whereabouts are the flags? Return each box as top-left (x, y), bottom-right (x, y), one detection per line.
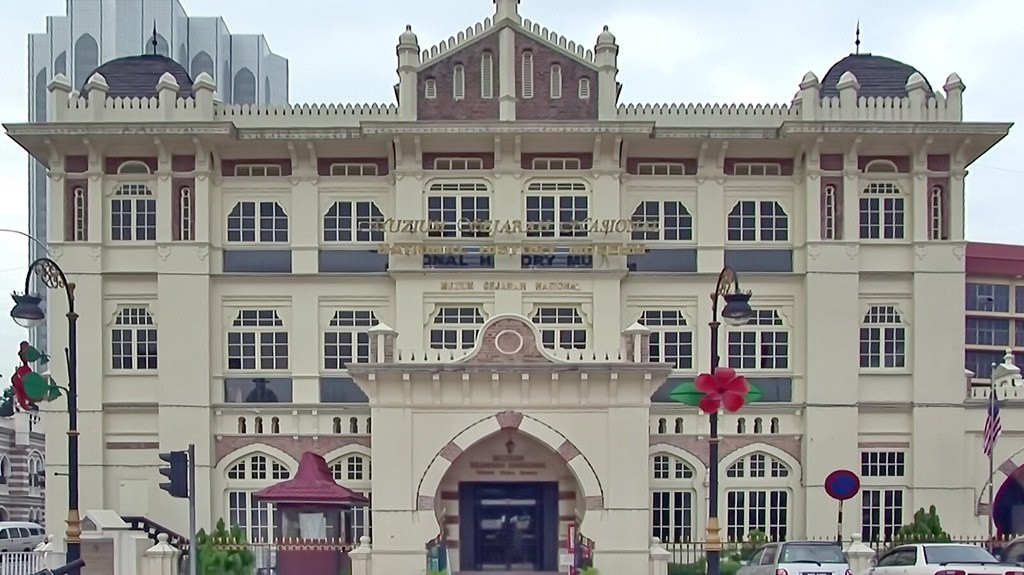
top-left (981, 387), bottom-right (1002, 460)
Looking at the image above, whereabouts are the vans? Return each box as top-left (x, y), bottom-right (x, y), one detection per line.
top-left (0, 520), bottom-right (48, 562)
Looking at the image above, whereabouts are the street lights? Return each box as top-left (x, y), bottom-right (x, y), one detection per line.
top-left (10, 256), bottom-right (83, 575)
top-left (702, 264), bottom-right (757, 575)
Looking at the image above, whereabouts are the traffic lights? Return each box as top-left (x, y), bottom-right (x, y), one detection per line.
top-left (158, 450), bottom-right (188, 498)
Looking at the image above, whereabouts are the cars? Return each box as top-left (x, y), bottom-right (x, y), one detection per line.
top-left (857, 535), bottom-right (1024, 575)
top-left (735, 540), bottom-right (853, 575)
